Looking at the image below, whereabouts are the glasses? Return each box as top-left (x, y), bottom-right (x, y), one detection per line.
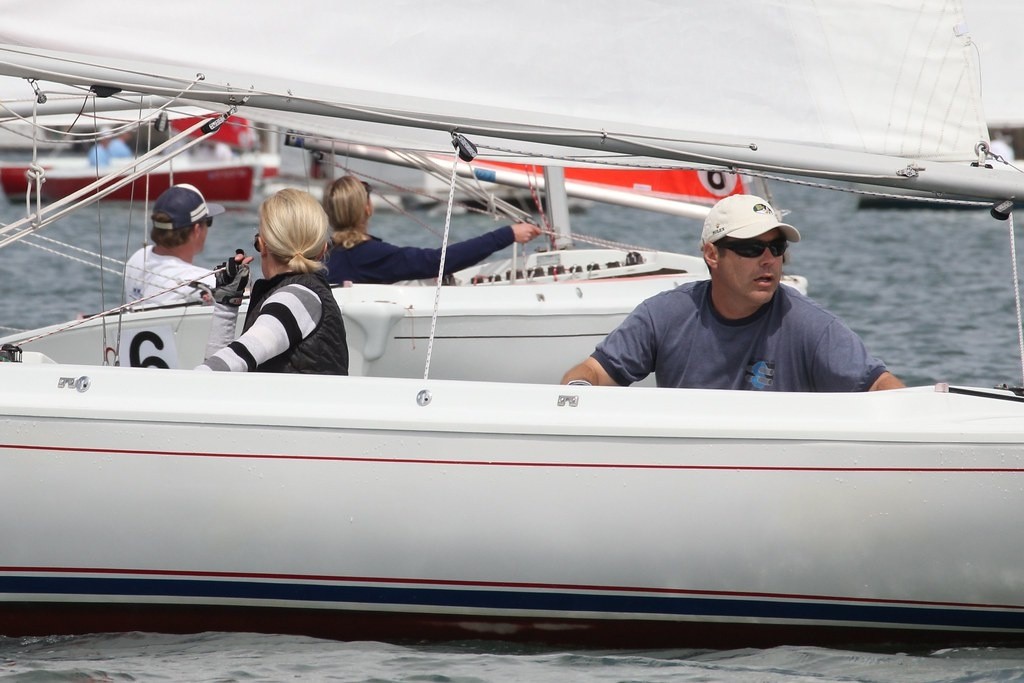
top-left (253, 233), bottom-right (268, 252)
top-left (360, 181), bottom-right (372, 206)
top-left (712, 238), bottom-right (789, 258)
top-left (199, 216), bottom-right (213, 227)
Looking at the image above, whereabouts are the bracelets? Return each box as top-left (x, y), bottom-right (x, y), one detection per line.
top-left (565, 379), bottom-right (592, 386)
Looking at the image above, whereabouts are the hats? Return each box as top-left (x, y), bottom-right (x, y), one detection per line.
top-left (151, 183), bottom-right (226, 230)
top-left (699, 193), bottom-right (801, 252)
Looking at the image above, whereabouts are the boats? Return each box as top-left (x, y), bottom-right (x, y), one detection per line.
top-left (1, 2), bottom-right (1024, 649)
top-left (2, 76), bottom-right (284, 211)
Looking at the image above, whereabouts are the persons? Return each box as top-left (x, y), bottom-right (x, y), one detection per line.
top-left (121, 183), bottom-right (250, 308)
top-left (192, 186), bottom-right (349, 376)
top-left (563, 192), bottom-right (907, 393)
top-left (322, 175), bottom-right (542, 285)
top-left (89, 124), bottom-right (136, 170)
